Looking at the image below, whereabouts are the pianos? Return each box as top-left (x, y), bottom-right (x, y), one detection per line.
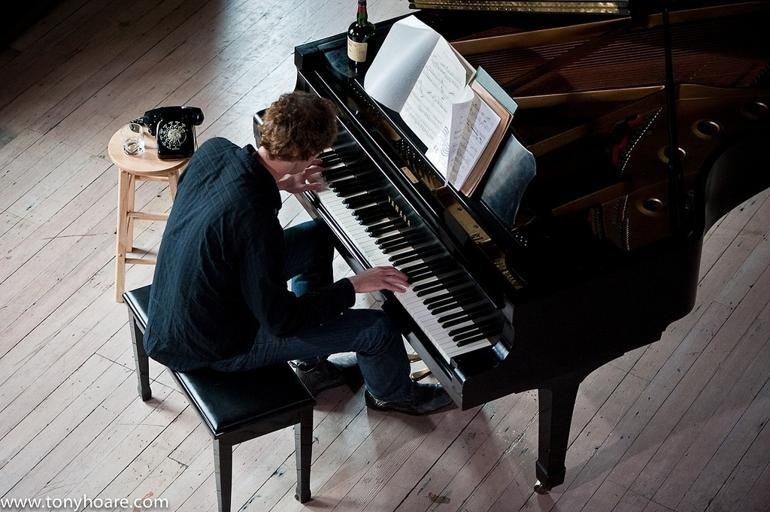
top-left (253, 1), bottom-right (769, 495)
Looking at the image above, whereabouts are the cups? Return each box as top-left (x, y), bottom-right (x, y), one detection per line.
top-left (121, 122), bottom-right (145, 157)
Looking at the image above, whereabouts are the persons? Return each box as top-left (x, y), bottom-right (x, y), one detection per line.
top-left (142, 92), bottom-right (457, 416)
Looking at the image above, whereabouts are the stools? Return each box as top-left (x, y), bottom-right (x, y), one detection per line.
top-left (106, 123), bottom-right (192, 304)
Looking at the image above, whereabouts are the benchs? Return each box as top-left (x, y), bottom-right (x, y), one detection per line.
top-left (122, 283), bottom-right (321, 511)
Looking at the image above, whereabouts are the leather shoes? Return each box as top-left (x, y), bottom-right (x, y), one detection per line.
top-left (364, 381), bottom-right (452, 418)
top-left (295, 356), bottom-right (359, 394)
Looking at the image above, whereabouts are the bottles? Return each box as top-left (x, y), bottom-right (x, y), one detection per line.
top-left (347, 0), bottom-right (376, 72)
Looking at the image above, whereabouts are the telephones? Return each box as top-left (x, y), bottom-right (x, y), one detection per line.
top-left (144, 105), bottom-right (204, 159)
